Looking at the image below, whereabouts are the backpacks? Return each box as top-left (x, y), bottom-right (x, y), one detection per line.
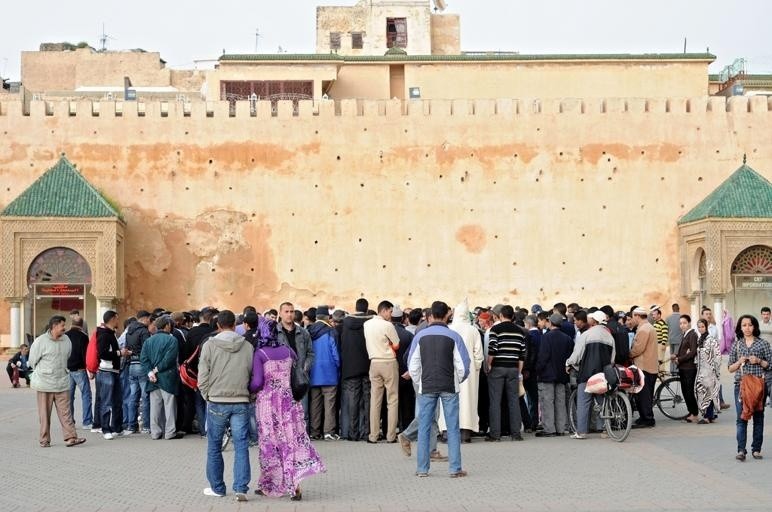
top-left (178, 343), bottom-right (200, 392)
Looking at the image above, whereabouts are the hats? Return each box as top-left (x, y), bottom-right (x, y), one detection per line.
top-left (587, 310), bottom-right (608, 325)
top-left (633, 307), bottom-right (648, 316)
top-left (316, 305), bottom-right (330, 316)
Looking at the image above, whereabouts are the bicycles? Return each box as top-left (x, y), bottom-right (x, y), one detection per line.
top-left (203, 409), bottom-right (231, 448)
top-left (628, 360), bottom-right (692, 421)
top-left (567, 366), bottom-right (633, 442)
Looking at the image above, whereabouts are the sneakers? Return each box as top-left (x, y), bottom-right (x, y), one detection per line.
top-left (448, 469), bottom-right (467, 478)
top-left (290, 487), bottom-right (302, 501)
top-left (254, 488), bottom-right (266, 496)
top-left (65, 436), bottom-right (86, 448)
top-left (398, 433), bottom-right (412, 456)
top-left (416, 468), bottom-right (429, 477)
top-left (235, 490), bottom-right (249, 502)
top-left (39, 438), bottom-right (51, 448)
top-left (751, 450), bottom-right (763, 460)
top-left (430, 449), bottom-right (451, 463)
top-left (735, 451), bottom-right (747, 461)
top-left (203, 488), bottom-right (226, 497)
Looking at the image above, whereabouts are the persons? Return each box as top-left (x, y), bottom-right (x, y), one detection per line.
top-left (197, 309), bottom-right (254, 501)
top-left (665, 303), bottom-right (771, 461)
top-left (275, 302), bottom-right (316, 432)
top-left (398, 310), bottom-right (449, 462)
top-left (6, 343), bottom-right (33, 387)
top-left (28, 317), bottom-right (86, 448)
top-left (406, 302), bottom-right (471, 478)
top-left (69, 298), bottom-right (668, 443)
top-left (249, 316), bottom-right (329, 499)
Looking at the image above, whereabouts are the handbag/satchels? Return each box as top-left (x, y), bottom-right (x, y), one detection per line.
top-left (290, 358), bottom-right (310, 401)
top-left (737, 373), bottom-right (767, 420)
